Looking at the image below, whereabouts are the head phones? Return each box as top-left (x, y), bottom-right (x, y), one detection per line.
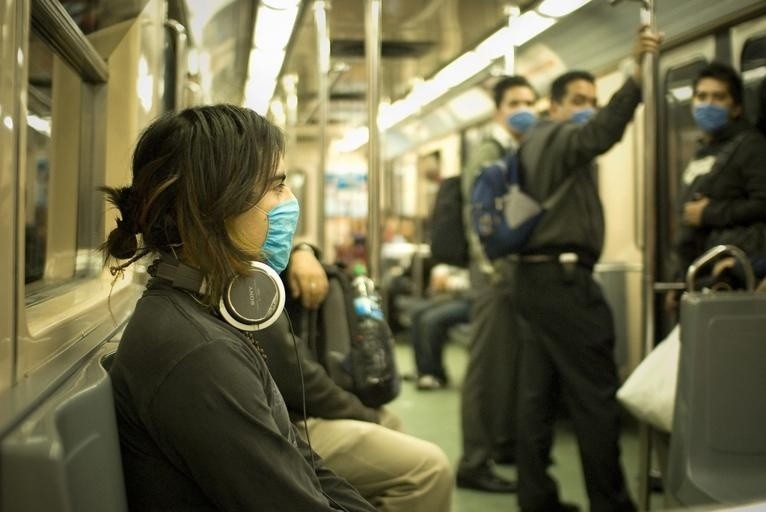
top-left (148, 256), bottom-right (286, 332)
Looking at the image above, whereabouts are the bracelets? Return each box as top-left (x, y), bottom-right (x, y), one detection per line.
top-left (291, 242), bottom-right (315, 250)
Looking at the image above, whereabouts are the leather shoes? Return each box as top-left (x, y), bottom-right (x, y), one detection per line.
top-left (455, 462), bottom-right (519, 494)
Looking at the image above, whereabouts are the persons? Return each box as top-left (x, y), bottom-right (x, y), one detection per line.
top-left (395, 260), bottom-right (472, 389)
top-left (99, 103), bottom-right (385, 511)
top-left (642, 63), bottom-right (766, 493)
top-left (508, 22), bottom-right (665, 511)
top-left (253, 235), bottom-right (454, 510)
top-left (449, 72), bottom-right (541, 494)
top-left (331, 207), bottom-right (433, 336)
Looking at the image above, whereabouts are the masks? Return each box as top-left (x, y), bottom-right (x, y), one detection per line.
top-left (691, 101), bottom-right (731, 131)
top-left (505, 108), bottom-right (536, 134)
top-left (230, 198), bottom-right (302, 279)
top-left (570, 108), bottom-right (597, 129)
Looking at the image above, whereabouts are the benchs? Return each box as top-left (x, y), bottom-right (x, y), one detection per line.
top-left (1, 291), bottom-right (139, 512)
top-left (389, 245), bottom-right (630, 366)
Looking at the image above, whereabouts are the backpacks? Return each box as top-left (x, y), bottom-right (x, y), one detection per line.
top-left (431, 175), bottom-right (471, 269)
top-left (311, 260), bottom-right (401, 407)
top-left (470, 117), bottom-right (579, 262)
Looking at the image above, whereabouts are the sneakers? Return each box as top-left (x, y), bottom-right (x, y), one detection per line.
top-left (401, 370), bottom-right (443, 391)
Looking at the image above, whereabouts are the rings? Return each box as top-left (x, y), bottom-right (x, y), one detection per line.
top-left (309, 282), bottom-right (317, 290)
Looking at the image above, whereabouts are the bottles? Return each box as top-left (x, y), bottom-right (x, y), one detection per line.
top-left (346, 262), bottom-right (400, 409)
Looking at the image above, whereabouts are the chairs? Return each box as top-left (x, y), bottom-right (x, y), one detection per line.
top-left (661, 290), bottom-right (766, 506)
